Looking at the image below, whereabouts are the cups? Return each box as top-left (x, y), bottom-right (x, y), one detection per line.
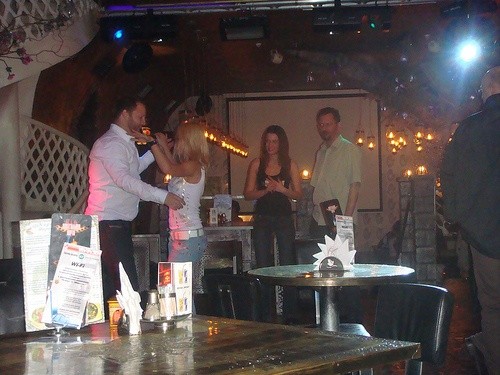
top-left (160, 293), bottom-right (176, 317)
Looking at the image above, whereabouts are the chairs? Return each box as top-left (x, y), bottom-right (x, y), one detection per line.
top-left (373, 283), bottom-right (455, 375)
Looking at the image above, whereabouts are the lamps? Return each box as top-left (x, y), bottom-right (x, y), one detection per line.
top-left (299, 169), bottom-right (311, 180)
top-left (355, 123), bottom-right (453, 192)
top-left (313, 14), bottom-right (364, 33)
top-left (368, 6), bottom-right (394, 32)
top-left (102, 12), bottom-right (274, 160)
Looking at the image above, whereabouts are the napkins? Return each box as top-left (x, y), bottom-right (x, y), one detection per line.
top-left (115, 261), bottom-right (143, 334)
top-left (311, 235), bottom-right (356, 271)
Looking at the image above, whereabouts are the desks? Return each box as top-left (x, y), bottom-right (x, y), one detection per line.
top-left (0, 314), bottom-right (422, 375)
top-left (247, 263), bottom-right (416, 332)
top-left (203, 220), bottom-right (256, 274)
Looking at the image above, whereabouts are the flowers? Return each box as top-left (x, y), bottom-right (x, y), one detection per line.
top-left (0, 0), bottom-right (74, 81)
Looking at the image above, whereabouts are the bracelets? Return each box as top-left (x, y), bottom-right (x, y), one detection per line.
top-left (147, 140), bottom-right (158, 147)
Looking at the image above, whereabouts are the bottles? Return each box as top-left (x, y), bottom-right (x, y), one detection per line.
top-left (143, 288), bottom-right (160, 320)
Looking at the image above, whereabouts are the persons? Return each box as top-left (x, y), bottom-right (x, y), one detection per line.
top-left (126, 119), bottom-right (211, 315)
top-left (308, 107), bottom-right (362, 264)
top-left (83, 97), bottom-right (186, 321)
top-left (243, 125), bottom-right (302, 326)
top-left (438, 66), bottom-right (500, 375)
